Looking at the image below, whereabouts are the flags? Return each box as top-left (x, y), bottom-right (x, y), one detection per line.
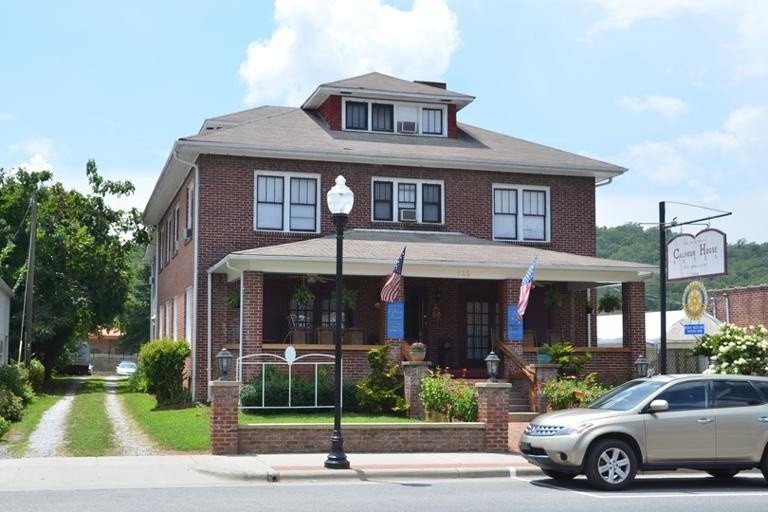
top-left (516, 255), bottom-right (537, 320)
top-left (380, 246), bottom-right (408, 304)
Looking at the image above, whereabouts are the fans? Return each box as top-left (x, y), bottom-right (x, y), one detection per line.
top-left (289, 273), bottom-right (338, 284)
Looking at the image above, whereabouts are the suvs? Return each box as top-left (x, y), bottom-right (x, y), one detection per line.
top-left (509, 371), bottom-right (768, 490)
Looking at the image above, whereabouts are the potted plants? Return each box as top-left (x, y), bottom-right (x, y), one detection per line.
top-left (409, 342), bottom-right (426, 360)
top-left (292, 285), bottom-right (314, 306)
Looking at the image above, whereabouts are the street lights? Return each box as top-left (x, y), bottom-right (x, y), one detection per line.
top-left (323, 172), bottom-right (355, 470)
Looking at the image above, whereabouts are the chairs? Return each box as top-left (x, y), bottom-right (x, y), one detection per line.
top-left (544, 326), bottom-right (565, 346)
top-left (523, 329), bottom-right (539, 348)
top-left (288, 325), bottom-right (313, 344)
top-left (316, 327), bottom-right (335, 344)
top-left (343, 326), bottom-right (366, 345)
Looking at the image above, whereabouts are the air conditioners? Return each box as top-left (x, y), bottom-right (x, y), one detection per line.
top-left (396, 120), bottom-right (417, 133)
top-left (398, 209), bottom-right (416, 222)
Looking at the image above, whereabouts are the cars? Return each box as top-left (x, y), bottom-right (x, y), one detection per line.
top-left (87, 363), bottom-right (94, 375)
top-left (115, 362), bottom-right (137, 377)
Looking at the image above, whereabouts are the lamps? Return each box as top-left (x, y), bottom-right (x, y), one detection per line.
top-left (634, 355), bottom-right (648, 377)
top-left (485, 352), bottom-right (500, 382)
top-left (216, 348), bottom-right (232, 381)
